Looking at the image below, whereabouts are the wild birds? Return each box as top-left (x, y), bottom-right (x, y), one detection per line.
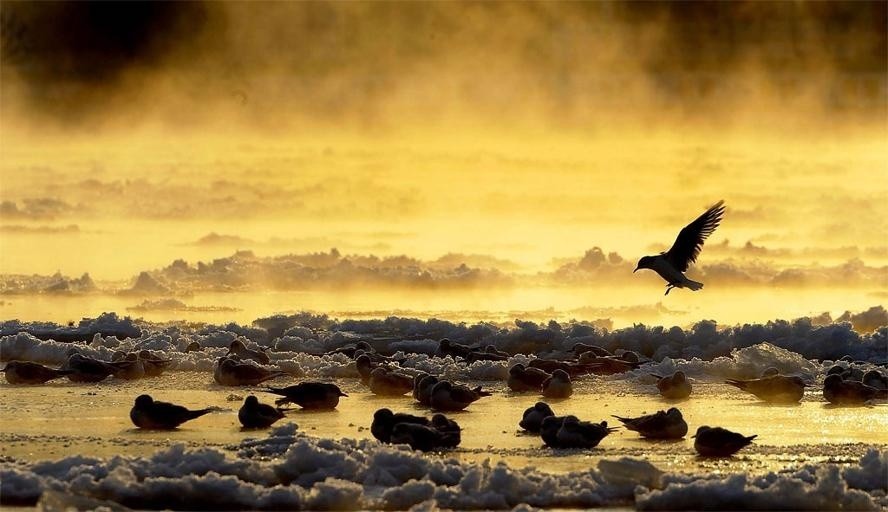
top-left (309, 341), bottom-right (417, 395)
top-left (691, 426), bottom-right (758, 457)
top-left (611, 408), bottom-right (687, 438)
top-left (130, 395), bottom-right (215, 429)
top-left (650, 371), bottom-right (692, 398)
top-left (214, 340), bottom-right (288, 386)
top-left (238, 396), bottom-right (287, 429)
top-left (435, 338), bottom-right (511, 367)
top-left (724, 374), bottom-right (815, 402)
top-left (818, 355), bottom-right (888, 405)
top-left (506, 343), bottom-right (652, 398)
top-left (372, 408), bottom-right (464, 452)
top-left (1, 349), bottom-right (172, 383)
top-left (519, 402), bottom-right (622, 450)
top-left (261, 382), bottom-right (349, 409)
top-left (182, 342), bottom-right (205, 354)
top-left (414, 373), bottom-right (492, 411)
top-left (633, 200), bottom-right (726, 295)
